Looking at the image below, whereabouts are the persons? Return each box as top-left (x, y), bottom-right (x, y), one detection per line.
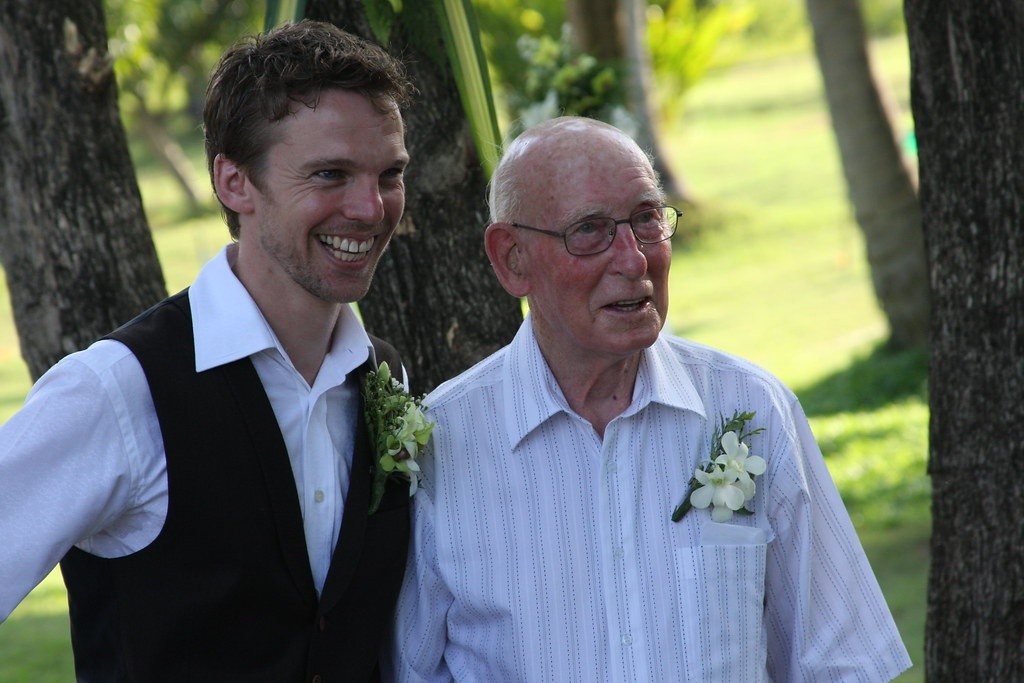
top-left (1, 20), bottom-right (420, 683)
top-left (393, 115), bottom-right (914, 683)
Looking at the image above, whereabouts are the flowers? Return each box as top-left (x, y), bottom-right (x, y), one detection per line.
top-left (362, 360), bottom-right (433, 496)
top-left (671, 408), bottom-right (768, 522)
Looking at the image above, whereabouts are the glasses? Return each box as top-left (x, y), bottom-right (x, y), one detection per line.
top-left (511, 204), bottom-right (684, 255)
